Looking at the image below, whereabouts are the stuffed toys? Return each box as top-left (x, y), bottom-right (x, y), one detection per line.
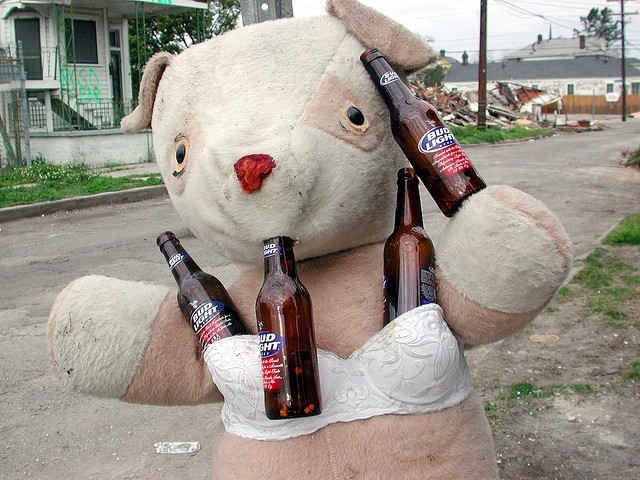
top-left (45, 0), bottom-right (573, 479)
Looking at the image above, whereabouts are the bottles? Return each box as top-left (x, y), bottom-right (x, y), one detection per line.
top-left (359, 47), bottom-right (487, 217)
top-left (255, 236), bottom-right (323, 420)
top-left (155, 230), bottom-right (251, 352)
top-left (381, 166), bottom-right (437, 327)
